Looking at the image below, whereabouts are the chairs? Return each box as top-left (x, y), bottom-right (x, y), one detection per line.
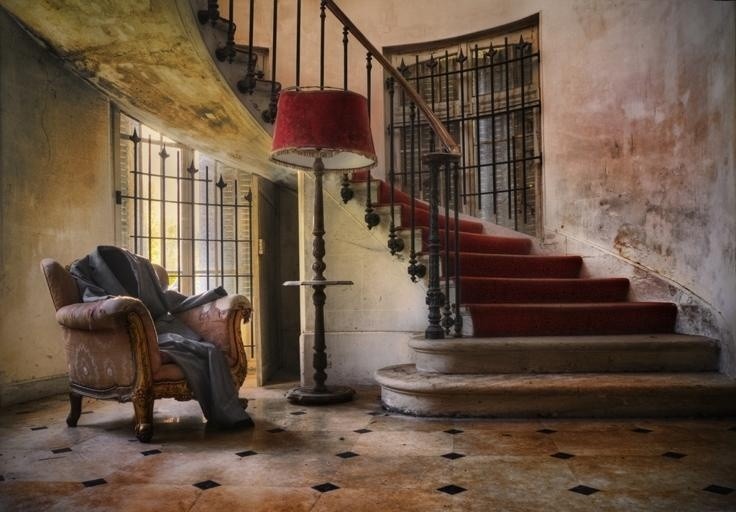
top-left (40, 257), bottom-right (254, 444)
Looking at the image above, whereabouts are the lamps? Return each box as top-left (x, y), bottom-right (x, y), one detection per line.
top-left (267, 86), bottom-right (378, 407)
